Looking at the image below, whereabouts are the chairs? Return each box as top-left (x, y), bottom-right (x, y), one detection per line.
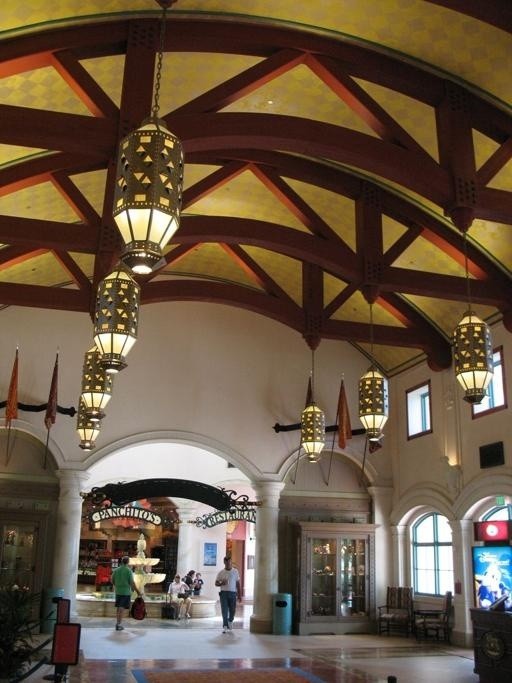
top-left (414, 591), bottom-right (451, 643)
top-left (377, 586), bottom-right (413, 638)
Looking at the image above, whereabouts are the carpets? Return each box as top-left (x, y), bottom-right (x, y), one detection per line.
top-left (131, 666), bottom-right (325, 683)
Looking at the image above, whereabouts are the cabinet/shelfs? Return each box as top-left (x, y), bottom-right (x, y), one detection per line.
top-left (78, 539), bottom-right (107, 569)
top-left (112, 540), bottom-right (137, 558)
top-left (0, 519), bottom-right (38, 588)
top-left (298, 520), bottom-right (381, 636)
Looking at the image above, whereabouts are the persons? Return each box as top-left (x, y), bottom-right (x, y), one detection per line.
top-left (195, 573), bottom-right (204, 596)
top-left (168, 573), bottom-right (192, 621)
top-left (215, 555), bottom-right (243, 633)
top-left (111, 555), bottom-right (144, 631)
top-left (182, 570), bottom-right (196, 590)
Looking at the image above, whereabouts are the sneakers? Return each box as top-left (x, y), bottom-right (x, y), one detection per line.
top-left (228, 621), bottom-right (233, 630)
top-left (222, 626), bottom-right (227, 633)
top-left (116, 625), bottom-right (123, 630)
top-left (184, 612), bottom-right (191, 618)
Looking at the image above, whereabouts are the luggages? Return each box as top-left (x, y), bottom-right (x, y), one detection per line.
top-left (162, 593), bottom-right (177, 619)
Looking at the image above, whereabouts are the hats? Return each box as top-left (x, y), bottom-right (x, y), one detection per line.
top-left (224, 556), bottom-right (231, 561)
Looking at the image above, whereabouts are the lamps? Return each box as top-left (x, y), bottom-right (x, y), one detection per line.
top-left (453, 232), bottom-right (493, 406)
top-left (111, 1), bottom-right (186, 275)
top-left (300, 349), bottom-right (326, 464)
top-left (81, 343), bottom-right (114, 421)
top-left (358, 303), bottom-right (390, 442)
top-left (75, 395), bottom-right (102, 450)
top-left (92, 260), bottom-right (141, 373)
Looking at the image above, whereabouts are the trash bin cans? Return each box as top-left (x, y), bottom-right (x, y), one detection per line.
top-left (272, 593), bottom-right (292, 635)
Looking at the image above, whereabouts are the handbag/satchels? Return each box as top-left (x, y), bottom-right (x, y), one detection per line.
top-left (131, 597), bottom-right (145, 620)
